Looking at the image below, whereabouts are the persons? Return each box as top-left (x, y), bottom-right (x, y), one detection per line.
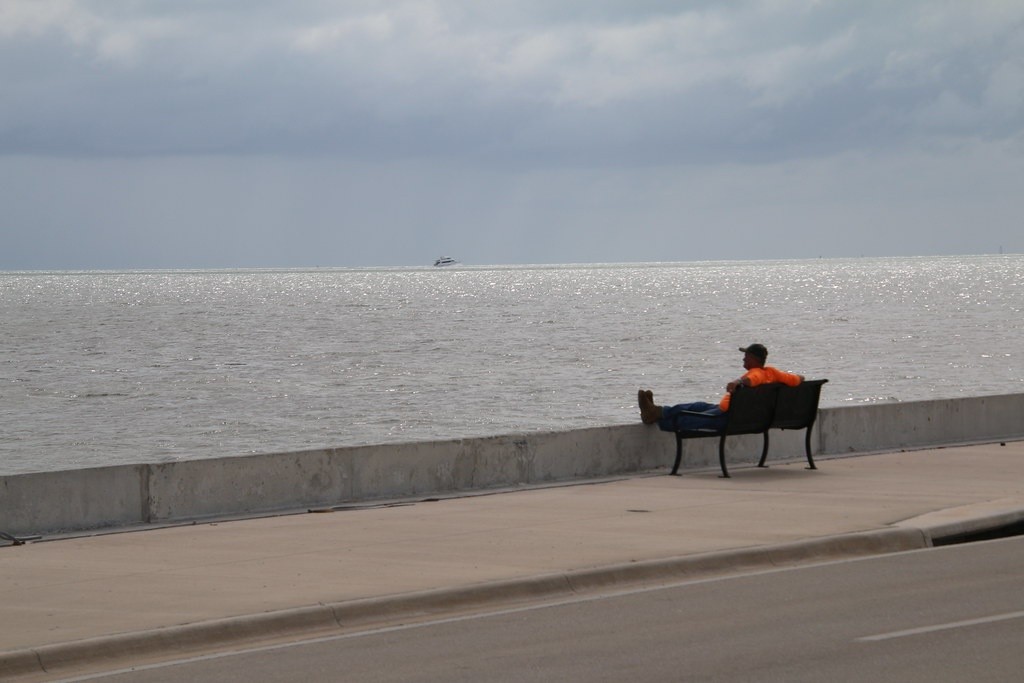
top-left (637, 343), bottom-right (806, 431)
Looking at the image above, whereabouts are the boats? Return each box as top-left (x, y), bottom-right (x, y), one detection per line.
top-left (433, 255), bottom-right (461, 267)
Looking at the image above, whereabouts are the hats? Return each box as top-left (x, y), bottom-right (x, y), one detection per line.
top-left (738, 343), bottom-right (767, 360)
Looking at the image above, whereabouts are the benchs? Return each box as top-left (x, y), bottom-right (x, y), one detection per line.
top-left (670, 378), bottom-right (829, 479)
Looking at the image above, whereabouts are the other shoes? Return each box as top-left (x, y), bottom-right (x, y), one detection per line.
top-left (638, 388), bottom-right (655, 425)
